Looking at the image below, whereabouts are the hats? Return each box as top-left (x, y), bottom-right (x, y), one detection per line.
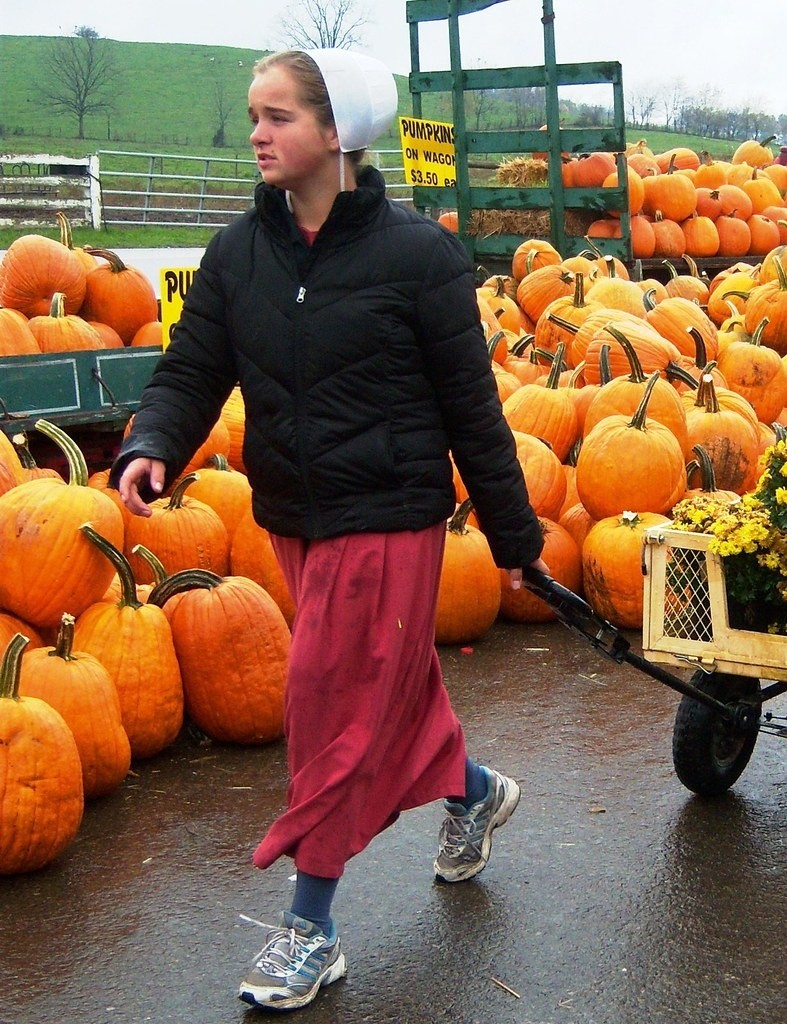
top-left (290, 48), bottom-right (399, 152)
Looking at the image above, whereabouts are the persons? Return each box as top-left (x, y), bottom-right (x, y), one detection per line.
top-left (105, 43), bottom-right (552, 1015)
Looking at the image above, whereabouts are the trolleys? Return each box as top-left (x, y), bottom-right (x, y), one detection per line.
top-left (523, 520), bottom-right (787, 797)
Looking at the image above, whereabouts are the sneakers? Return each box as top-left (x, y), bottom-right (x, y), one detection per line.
top-left (239, 911), bottom-right (348, 1009)
top-left (433, 765), bottom-right (522, 883)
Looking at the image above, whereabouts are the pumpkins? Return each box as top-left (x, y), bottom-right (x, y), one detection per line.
top-left (1, 136), bottom-right (787, 867)
